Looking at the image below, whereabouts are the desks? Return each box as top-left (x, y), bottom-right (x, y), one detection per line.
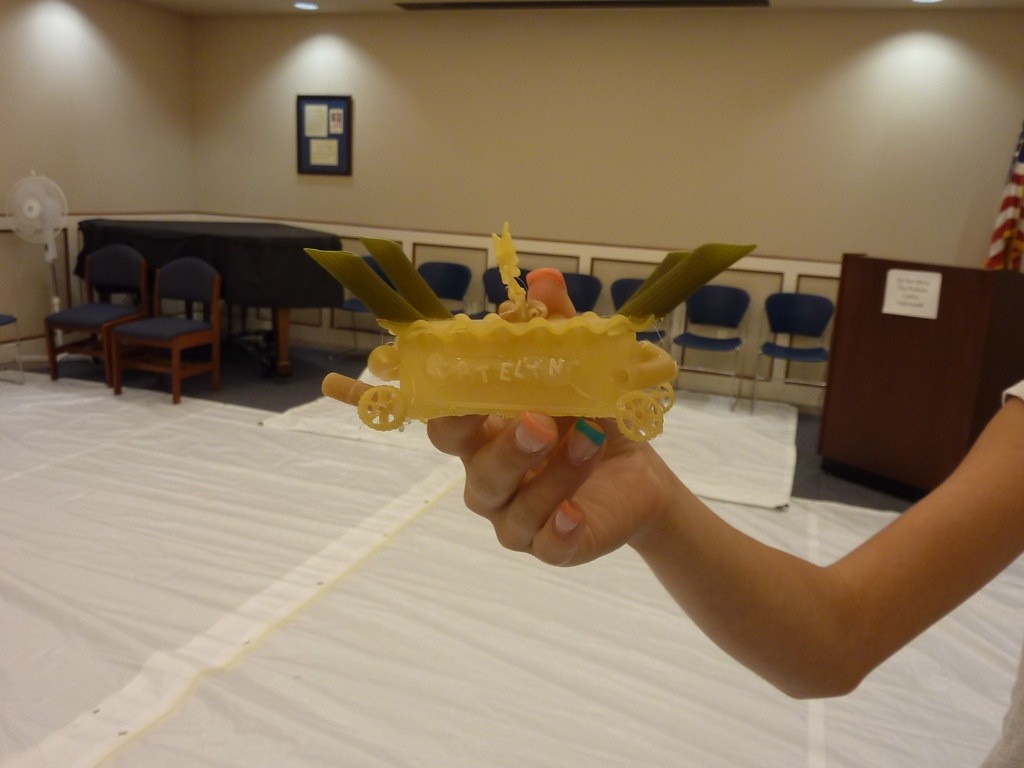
top-left (72, 220), bottom-right (342, 367)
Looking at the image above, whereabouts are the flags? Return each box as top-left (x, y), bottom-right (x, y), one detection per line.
top-left (983, 120), bottom-right (1024, 270)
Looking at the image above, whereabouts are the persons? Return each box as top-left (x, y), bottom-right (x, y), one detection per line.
top-left (425, 269), bottom-right (1024, 768)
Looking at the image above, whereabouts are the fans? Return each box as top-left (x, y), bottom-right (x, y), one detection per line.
top-left (5, 174), bottom-right (91, 362)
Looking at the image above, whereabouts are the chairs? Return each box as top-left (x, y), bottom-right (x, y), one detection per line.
top-left (42, 245), bottom-right (149, 386)
top-left (469, 267), bottom-right (532, 319)
top-left (562, 273), bottom-right (603, 311)
top-left (672, 285), bottom-right (751, 412)
top-left (416, 261), bottom-right (472, 316)
top-left (750, 291), bottom-right (835, 412)
top-left (0, 313), bottom-right (24, 386)
top-left (339, 255), bottom-right (395, 349)
top-left (112, 258), bottom-right (221, 405)
top-left (609, 280), bottom-right (672, 345)
